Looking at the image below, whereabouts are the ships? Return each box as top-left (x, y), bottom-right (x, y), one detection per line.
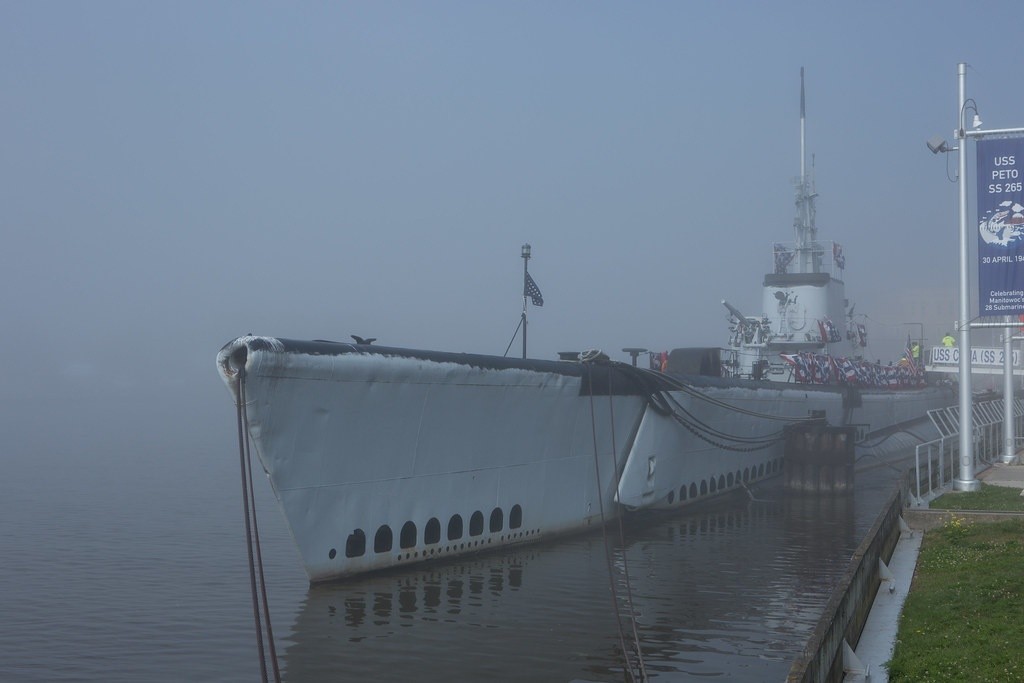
top-left (214, 64), bottom-right (960, 581)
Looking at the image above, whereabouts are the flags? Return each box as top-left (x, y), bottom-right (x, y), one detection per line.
top-left (906, 334), bottom-right (915, 373)
top-left (779, 352), bottom-right (925, 388)
top-left (525, 272), bottom-right (544, 306)
top-left (833, 244), bottom-right (845, 268)
top-left (819, 320), bottom-right (842, 343)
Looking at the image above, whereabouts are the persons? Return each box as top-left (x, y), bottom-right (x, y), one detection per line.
top-left (942, 332), bottom-right (955, 346)
top-left (912, 341), bottom-right (919, 363)
